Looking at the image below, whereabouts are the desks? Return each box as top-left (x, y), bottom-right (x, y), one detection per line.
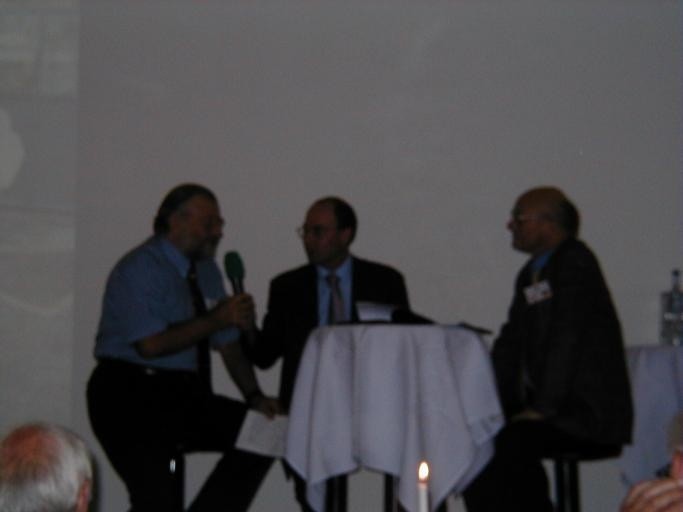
top-left (281, 322), bottom-right (506, 512)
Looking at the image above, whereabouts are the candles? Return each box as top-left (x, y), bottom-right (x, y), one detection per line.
top-left (417, 462), bottom-right (429, 512)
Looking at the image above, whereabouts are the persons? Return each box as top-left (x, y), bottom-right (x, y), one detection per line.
top-left (0, 422), bottom-right (94, 511)
top-left (619, 411), bottom-right (683, 511)
top-left (86, 182), bottom-right (277, 511)
top-left (242, 196), bottom-right (435, 512)
top-left (459, 185), bottom-right (634, 511)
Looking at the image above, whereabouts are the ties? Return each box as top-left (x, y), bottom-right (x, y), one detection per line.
top-left (185, 261), bottom-right (209, 371)
top-left (325, 275), bottom-right (344, 326)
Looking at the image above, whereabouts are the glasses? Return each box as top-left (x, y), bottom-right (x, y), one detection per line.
top-left (297, 223), bottom-right (339, 238)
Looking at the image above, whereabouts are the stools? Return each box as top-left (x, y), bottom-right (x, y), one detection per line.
top-left (544, 446), bottom-right (622, 512)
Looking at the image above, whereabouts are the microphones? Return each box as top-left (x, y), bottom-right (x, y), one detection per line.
top-left (224, 251), bottom-right (245, 296)
